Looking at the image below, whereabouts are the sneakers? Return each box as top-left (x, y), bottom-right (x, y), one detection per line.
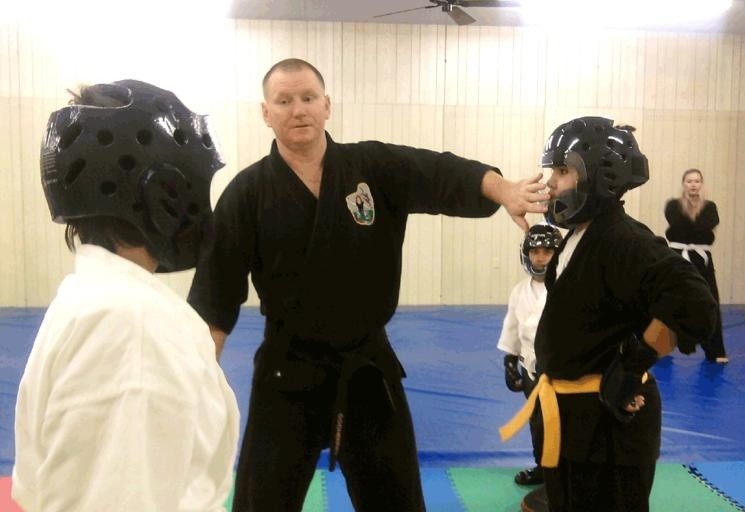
top-left (514, 466), bottom-right (544, 485)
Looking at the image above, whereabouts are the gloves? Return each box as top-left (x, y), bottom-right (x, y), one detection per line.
top-left (503, 355), bottom-right (525, 392)
top-left (597, 331), bottom-right (652, 418)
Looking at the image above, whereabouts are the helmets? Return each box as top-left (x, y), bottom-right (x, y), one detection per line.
top-left (518, 224), bottom-right (565, 284)
top-left (38, 78), bottom-right (227, 272)
top-left (538, 115), bottom-right (649, 230)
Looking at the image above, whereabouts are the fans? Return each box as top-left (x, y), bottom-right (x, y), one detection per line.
top-left (373, 0), bottom-right (520, 25)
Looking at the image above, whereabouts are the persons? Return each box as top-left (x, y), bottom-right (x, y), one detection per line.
top-left (186, 58), bottom-right (554, 511)
top-left (661, 166), bottom-right (730, 366)
top-left (493, 222), bottom-right (564, 486)
top-left (8, 75), bottom-right (241, 511)
top-left (532, 115), bottom-right (721, 512)
